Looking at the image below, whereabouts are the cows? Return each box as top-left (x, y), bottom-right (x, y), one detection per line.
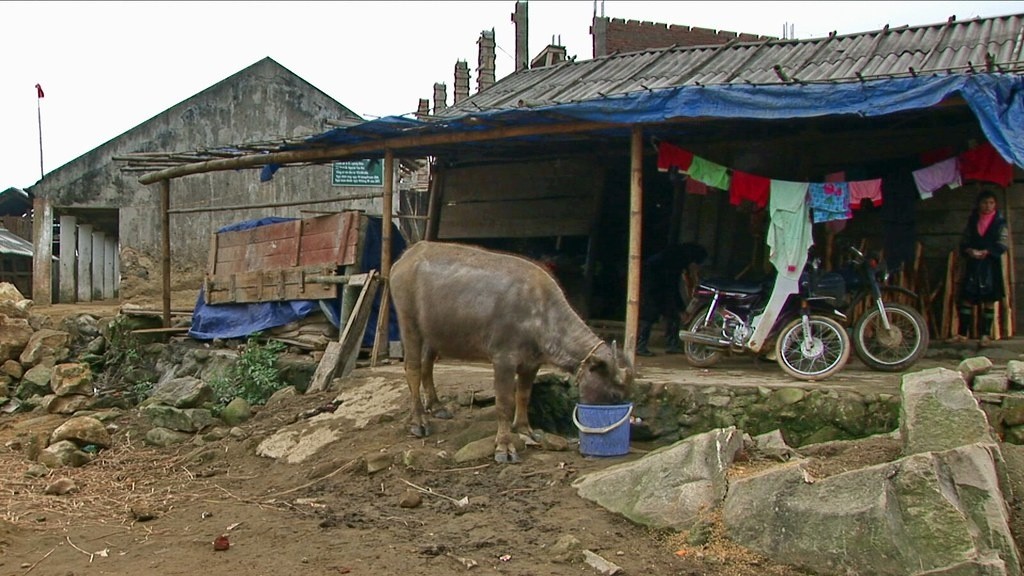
top-left (390, 239), bottom-right (633, 464)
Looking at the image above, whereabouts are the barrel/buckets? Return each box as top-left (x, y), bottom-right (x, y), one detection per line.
top-left (571, 402), bottom-right (635, 457)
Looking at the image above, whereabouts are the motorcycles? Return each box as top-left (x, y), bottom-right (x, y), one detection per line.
top-left (675, 238), bottom-right (852, 382)
top-left (750, 234), bottom-right (931, 370)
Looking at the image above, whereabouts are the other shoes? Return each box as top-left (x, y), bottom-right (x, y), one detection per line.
top-left (944, 335), bottom-right (968, 344)
top-left (979, 336), bottom-right (991, 347)
top-left (637, 349), bottom-right (654, 357)
top-left (666, 347), bottom-right (684, 355)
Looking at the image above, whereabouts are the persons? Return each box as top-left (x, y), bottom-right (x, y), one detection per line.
top-left (638, 242), bottom-right (707, 357)
top-left (959, 191), bottom-right (1009, 340)
